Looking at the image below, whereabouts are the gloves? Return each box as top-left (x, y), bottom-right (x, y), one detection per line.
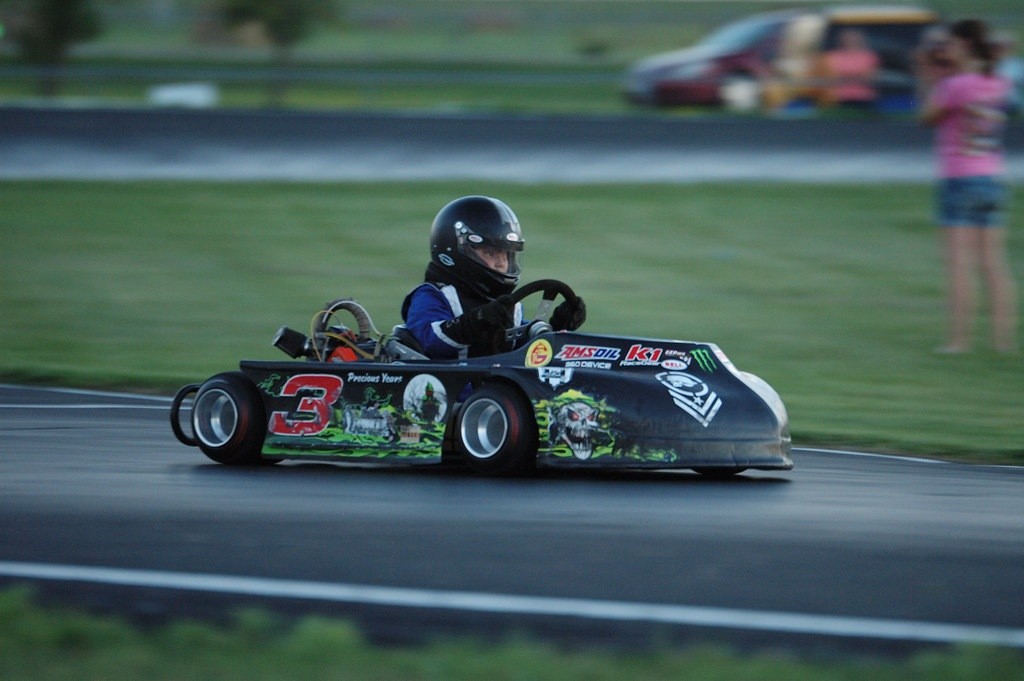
top-left (459, 292), bottom-right (514, 344)
top-left (550, 296), bottom-right (587, 332)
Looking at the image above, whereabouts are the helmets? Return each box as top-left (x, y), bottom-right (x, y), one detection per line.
top-left (430, 196), bottom-right (525, 297)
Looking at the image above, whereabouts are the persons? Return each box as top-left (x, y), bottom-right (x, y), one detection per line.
top-left (773, 14), bottom-right (882, 111)
top-left (401, 196), bottom-right (587, 361)
top-left (914, 17), bottom-right (1024, 355)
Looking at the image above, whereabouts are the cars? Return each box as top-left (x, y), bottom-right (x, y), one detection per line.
top-left (754, 6), bottom-right (948, 113)
top-left (617, 9), bottom-right (801, 111)
